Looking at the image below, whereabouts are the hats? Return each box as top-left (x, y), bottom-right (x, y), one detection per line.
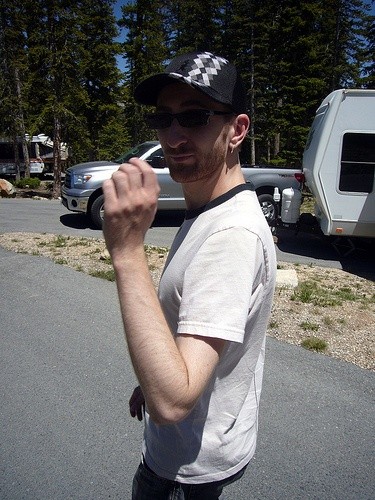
top-left (133, 51), bottom-right (246, 113)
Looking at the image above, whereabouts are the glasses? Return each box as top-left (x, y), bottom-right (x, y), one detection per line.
top-left (144, 109), bottom-right (241, 129)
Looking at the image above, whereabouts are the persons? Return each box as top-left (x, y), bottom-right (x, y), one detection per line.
top-left (102, 49), bottom-right (277, 500)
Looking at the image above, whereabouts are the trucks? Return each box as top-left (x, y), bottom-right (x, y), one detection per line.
top-left (303, 89), bottom-right (375, 238)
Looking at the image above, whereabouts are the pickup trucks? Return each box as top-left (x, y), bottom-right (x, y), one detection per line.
top-left (61, 140), bottom-right (302, 230)
